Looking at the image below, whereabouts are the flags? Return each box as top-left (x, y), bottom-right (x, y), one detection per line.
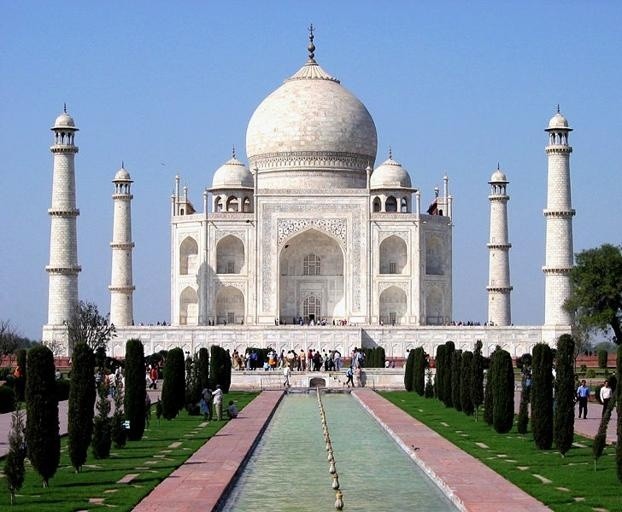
top-left (426, 198), bottom-right (438, 215)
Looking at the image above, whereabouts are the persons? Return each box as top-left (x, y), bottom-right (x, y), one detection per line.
top-left (222, 400), bottom-right (238, 419)
top-left (385, 358), bottom-right (390, 368)
top-left (201, 383), bottom-right (214, 422)
top-left (282, 365), bottom-right (291, 386)
top-left (526, 375), bottom-right (532, 402)
top-left (389, 356), bottom-right (397, 368)
top-left (353, 364), bottom-right (363, 387)
top-left (157, 320), bottom-right (160, 326)
top-left (210, 384), bottom-right (224, 421)
top-left (441, 319), bottom-right (498, 326)
top-left (162, 320), bottom-right (167, 326)
top-left (55, 369), bottom-right (62, 380)
top-left (186, 351), bottom-right (192, 359)
top-left (93, 363), bottom-right (160, 400)
top-left (600, 380), bottom-right (612, 418)
top-left (391, 320), bottom-right (396, 326)
top-left (380, 319), bottom-right (384, 326)
top-left (225, 345), bottom-right (368, 371)
top-left (273, 314), bottom-right (347, 326)
top-left (403, 349), bottom-right (436, 368)
top-left (576, 379), bottom-right (591, 419)
top-left (345, 365), bottom-right (355, 388)
